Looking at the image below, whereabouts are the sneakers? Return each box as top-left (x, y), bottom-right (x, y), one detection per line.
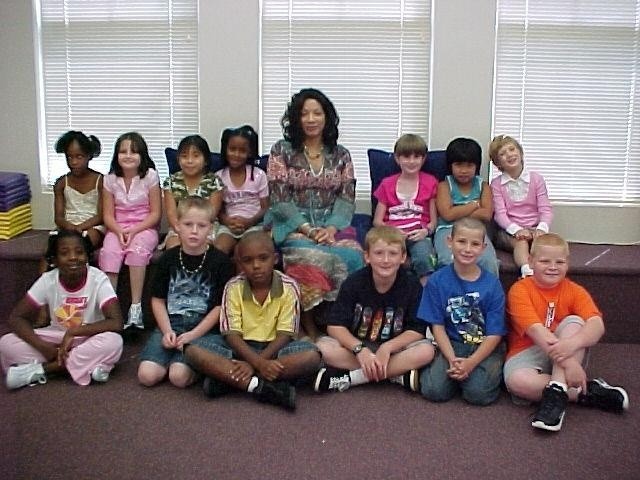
top-left (91, 367), bottom-right (109, 383)
top-left (6, 364), bottom-right (47, 390)
top-left (124, 304), bottom-right (144, 330)
top-left (531, 384), bottom-right (569, 432)
top-left (203, 377), bottom-right (296, 409)
top-left (314, 368), bottom-right (351, 393)
top-left (390, 369), bottom-right (419, 392)
top-left (576, 377), bottom-right (629, 415)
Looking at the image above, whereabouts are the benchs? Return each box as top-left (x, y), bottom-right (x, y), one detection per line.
top-left (0, 228), bottom-right (640, 345)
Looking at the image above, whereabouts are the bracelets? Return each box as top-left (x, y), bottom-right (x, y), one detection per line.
top-left (307, 228), bottom-right (316, 237)
top-left (473, 200), bottom-right (481, 208)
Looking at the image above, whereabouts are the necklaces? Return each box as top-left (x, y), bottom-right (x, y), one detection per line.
top-left (179, 247), bottom-right (207, 274)
top-left (303, 143), bottom-right (324, 178)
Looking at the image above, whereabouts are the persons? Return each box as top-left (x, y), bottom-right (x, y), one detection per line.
top-left (488, 135), bottom-right (553, 279)
top-left (371, 133), bottom-right (440, 288)
top-left (503, 234), bottom-right (629, 432)
top-left (416, 216), bottom-right (507, 406)
top-left (98, 132), bottom-right (162, 331)
top-left (0, 230), bottom-right (124, 390)
top-left (262, 88), bottom-right (367, 339)
top-left (214, 125), bottom-right (270, 256)
top-left (183, 230), bottom-right (322, 411)
top-left (34, 131), bottom-right (103, 325)
top-left (432, 138), bottom-right (500, 280)
top-left (314, 225), bottom-right (435, 395)
top-left (163, 135), bottom-right (225, 250)
top-left (137, 199), bottom-right (233, 389)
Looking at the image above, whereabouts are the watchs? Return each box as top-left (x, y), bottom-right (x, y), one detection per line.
top-left (425, 226), bottom-right (432, 237)
top-left (352, 343), bottom-right (367, 356)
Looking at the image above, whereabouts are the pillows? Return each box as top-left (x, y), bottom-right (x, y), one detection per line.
top-left (165, 147), bottom-right (269, 177)
top-left (367, 148), bottom-right (446, 217)
